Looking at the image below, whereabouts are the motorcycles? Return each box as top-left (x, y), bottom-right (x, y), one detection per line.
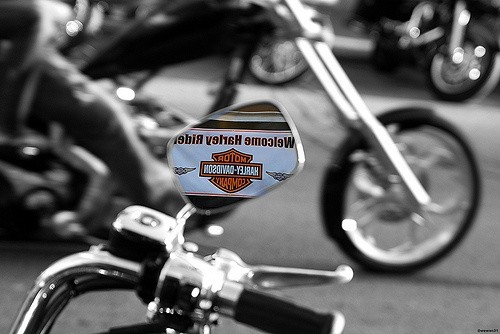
top-left (0, 0), bottom-right (500, 334)
